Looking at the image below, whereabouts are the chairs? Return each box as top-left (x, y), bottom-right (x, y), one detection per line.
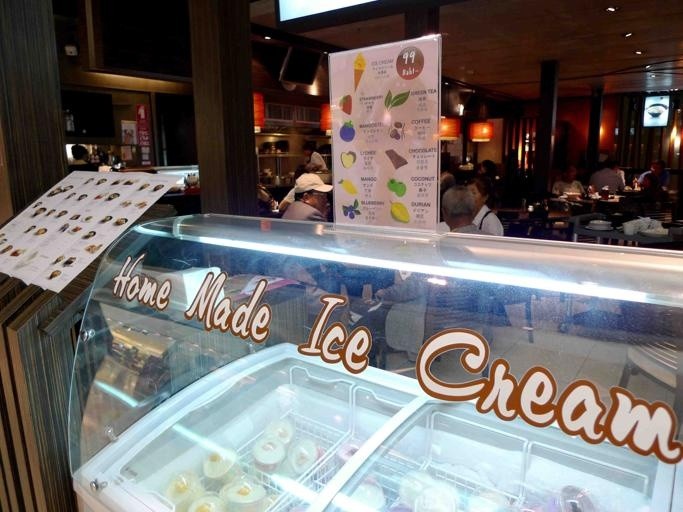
top-left (618, 334), bottom-right (682, 425)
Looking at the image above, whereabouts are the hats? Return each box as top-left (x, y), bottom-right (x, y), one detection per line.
top-left (295, 173), bottom-right (334, 195)
top-left (71, 145), bottom-right (88, 159)
top-left (303, 143), bottom-right (318, 151)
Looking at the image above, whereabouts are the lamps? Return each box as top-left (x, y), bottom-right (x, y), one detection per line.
top-left (440, 119), bottom-right (460, 141)
top-left (469, 123), bottom-right (494, 142)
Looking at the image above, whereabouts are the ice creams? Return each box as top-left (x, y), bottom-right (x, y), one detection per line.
top-left (527, 485), bottom-right (594, 512)
top-left (251, 439), bottom-right (285, 482)
top-left (353, 54), bottom-right (366, 91)
top-left (335, 443), bottom-right (358, 469)
top-left (278, 444), bottom-right (311, 479)
top-left (265, 420), bottom-right (298, 448)
top-left (222, 484), bottom-right (266, 512)
top-left (202, 448), bottom-right (242, 485)
top-left (296, 439), bottom-right (327, 464)
top-left (164, 470), bottom-right (206, 512)
top-left (186, 495), bottom-right (227, 512)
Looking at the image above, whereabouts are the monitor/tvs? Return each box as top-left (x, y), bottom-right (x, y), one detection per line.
top-left (274, 0), bottom-right (458, 35)
top-left (277, 47), bottom-right (322, 85)
top-left (642, 96), bottom-right (669, 127)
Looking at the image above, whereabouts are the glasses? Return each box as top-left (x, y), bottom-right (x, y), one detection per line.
top-left (313, 192), bottom-right (328, 200)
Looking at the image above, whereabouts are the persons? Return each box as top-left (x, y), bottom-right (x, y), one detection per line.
top-left (387, 262), bottom-right (453, 363)
top-left (635, 173), bottom-right (666, 207)
top-left (612, 162), bottom-right (626, 186)
top-left (278, 167), bottom-right (306, 209)
top-left (374, 184), bottom-right (494, 367)
top-left (441, 156), bottom-right (454, 190)
top-left (551, 161), bottom-right (584, 194)
top-left (304, 143), bottom-right (327, 171)
top-left (632, 160), bottom-right (670, 184)
top-left (589, 150), bottom-right (625, 189)
top-left (266, 173), bottom-right (347, 332)
top-left (476, 159), bottom-right (503, 200)
top-left (460, 181), bottom-right (503, 235)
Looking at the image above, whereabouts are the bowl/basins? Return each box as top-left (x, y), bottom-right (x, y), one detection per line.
top-left (588, 220), bottom-right (612, 231)
top-left (646, 103), bottom-right (669, 118)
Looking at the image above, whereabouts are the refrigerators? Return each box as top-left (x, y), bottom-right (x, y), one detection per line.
top-left (67, 339), bottom-right (680, 512)
